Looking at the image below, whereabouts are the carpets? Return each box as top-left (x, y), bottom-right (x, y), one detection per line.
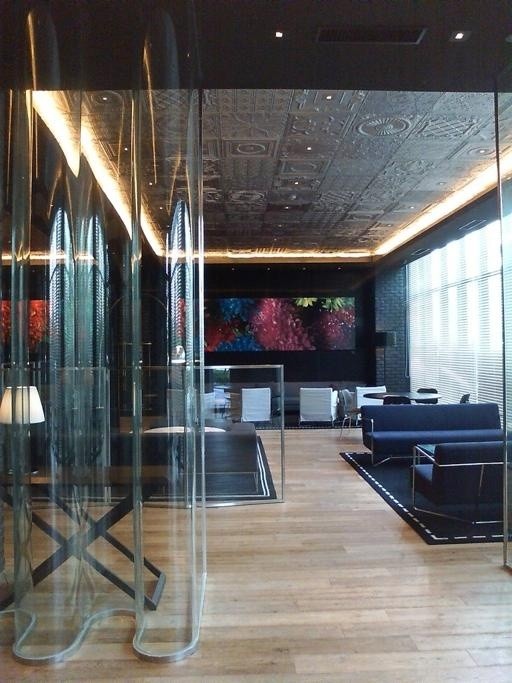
top-left (29, 435), bottom-right (277, 502)
top-left (340, 452), bottom-right (511, 545)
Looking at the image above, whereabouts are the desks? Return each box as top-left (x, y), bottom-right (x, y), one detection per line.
top-left (0, 467), bottom-right (171, 611)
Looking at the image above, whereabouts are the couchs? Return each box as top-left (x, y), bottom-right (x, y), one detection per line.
top-left (110, 422), bottom-right (257, 495)
top-left (411, 442), bottom-right (512, 524)
top-left (360, 404), bottom-right (511, 467)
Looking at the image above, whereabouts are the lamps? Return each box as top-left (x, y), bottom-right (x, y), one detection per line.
top-left (0, 386), bottom-right (46, 475)
top-left (375, 329), bottom-right (396, 391)
